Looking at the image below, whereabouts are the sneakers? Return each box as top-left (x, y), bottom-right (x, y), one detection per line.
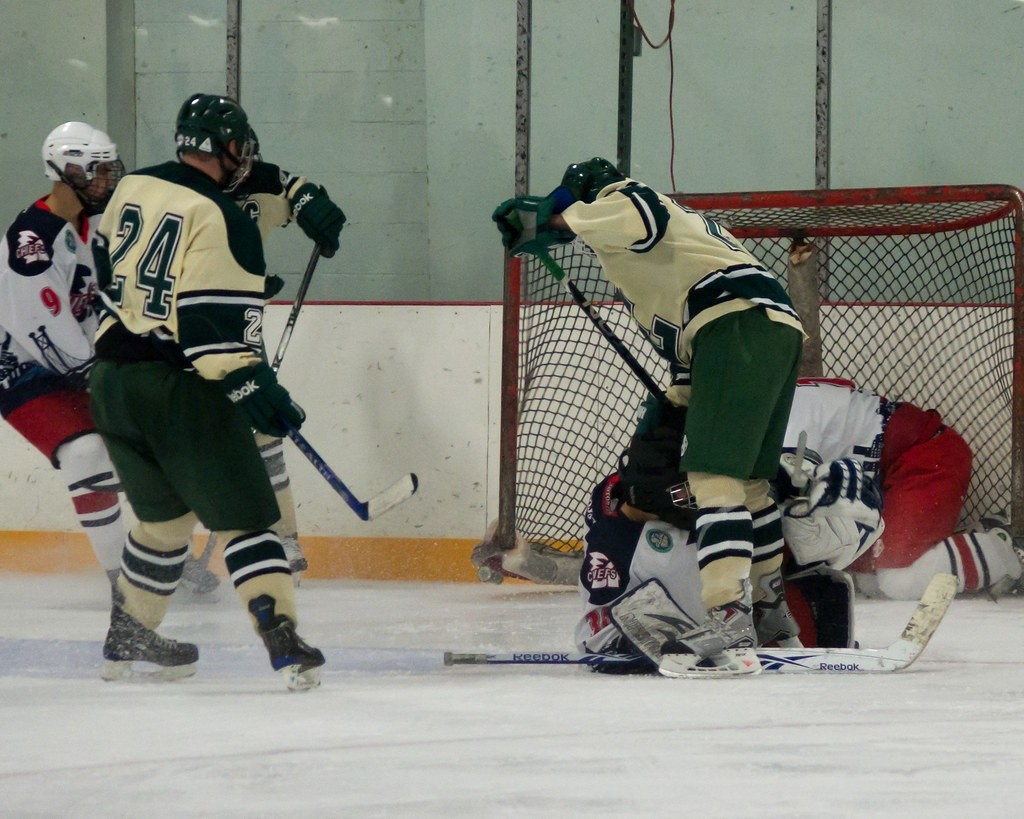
top-left (248, 593), bottom-right (325, 690)
top-left (280, 531), bottom-right (308, 591)
top-left (752, 567), bottom-right (806, 647)
top-left (657, 576), bottom-right (761, 678)
top-left (99, 585), bottom-right (199, 680)
top-left (179, 554), bottom-right (220, 602)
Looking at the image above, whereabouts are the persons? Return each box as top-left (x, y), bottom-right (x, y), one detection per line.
top-left (0, 121), bottom-right (220, 601)
top-left (580, 446), bottom-right (886, 655)
top-left (90, 92), bottom-right (345, 680)
top-left (771, 376), bottom-right (1021, 599)
top-left (492, 156), bottom-right (805, 678)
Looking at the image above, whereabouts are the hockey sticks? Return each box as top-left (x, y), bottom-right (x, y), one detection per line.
top-left (525, 246), bottom-right (681, 425)
top-left (283, 428), bottom-right (421, 521)
top-left (177, 242), bottom-right (325, 593)
top-left (442, 569), bottom-right (962, 677)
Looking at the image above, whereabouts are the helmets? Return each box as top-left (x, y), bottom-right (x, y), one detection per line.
top-left (175, 92), bottom-right (247, 180)
top-left (42, 122), bottom-right (117, 183)
top-left (245, 123), bottom-right (260, 156)
top-left (561, 156), bottom-right (625, 204)
